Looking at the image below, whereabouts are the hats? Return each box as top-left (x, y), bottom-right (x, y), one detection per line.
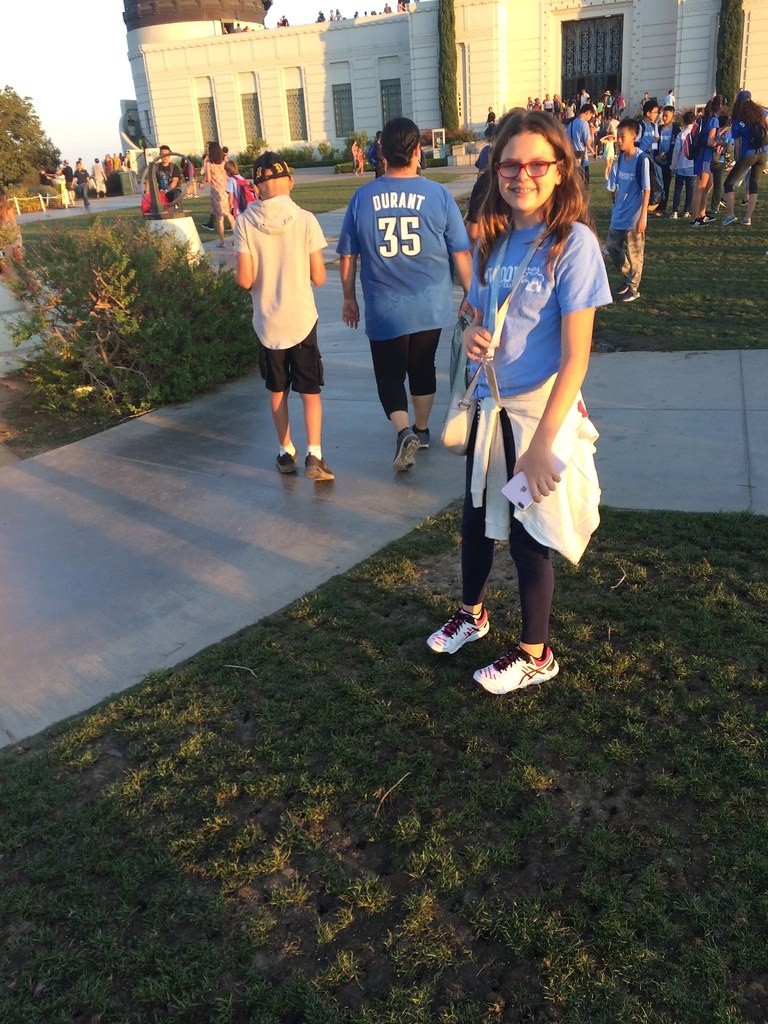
top-left (736, 91), bottom-right (752, 102)
top-left (252, 151), bottom-right (291, 187)
top-left (604, 91), bottom-right (611, 95)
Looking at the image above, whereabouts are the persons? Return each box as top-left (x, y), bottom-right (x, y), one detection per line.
top-left (181, 157), bottom-right (199, 198)
top-left (634, 91), bottom-right (768, 226)
top-left (45, 158), bottom-right (92, 206)
top-left (127, 113), bottom-right (136, 141)
top-left (234, 23), bottom-right (242, 32)
top-left (606, 118), bottom-right (650, 303)
top-left (92, 151), bottom-right (132, 199)
top-left (144, 145), bottom-right (182, 209)
top-left (138, 137), bottom-right (147, 149)
top-left (316, 2), bottom-right (406, 23)
top-left (426, 107), bottom-right (613, 695)
top-left (465, 89), bottom-right (627, 256)
top-left (227, 15), bottom-right (289, 33)
top-left (235, 151), bottom-right (334, 482)
top-left (336, 118), bottom-right (473, 472)
top-left (224, 160), bottom-right (247, 220)
top-left (199, 142), bottom-right (235, 248)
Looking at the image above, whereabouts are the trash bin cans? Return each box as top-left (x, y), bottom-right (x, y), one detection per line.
top-left (118, 170), bottom-right (139, 195)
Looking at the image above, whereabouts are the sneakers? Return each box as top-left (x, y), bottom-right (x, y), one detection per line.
top-left (655, 197), bottom-right (758, 227)
top-left (411, 424), bottom-right (431, 449)
top-left (619, 291), bottom-right (640, 301)
top-left (392, 427), bottom-right (420, 472)
top-left (424, 607), bottom-right (490, 655)
top-left (276, 449), bottom-right (298, 473)
top-left (617, 283), bottom-right (628, 295)
top-left (304, 452), bottom-right (334, 481)
top-left (472, 643), bottom-right (559, 695)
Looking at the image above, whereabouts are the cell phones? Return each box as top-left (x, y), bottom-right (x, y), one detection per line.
top-left (502, 454), bottom-right (566, 511)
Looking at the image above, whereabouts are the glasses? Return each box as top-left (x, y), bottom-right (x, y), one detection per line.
top-left (495, 159), bottom-right (561, 178)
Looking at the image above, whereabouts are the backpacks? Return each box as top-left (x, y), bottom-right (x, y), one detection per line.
top-left (684, 115), bottom-right (718, 160)
top-left (231, 175), bottom-right (255, 211)
top-left (369, 145), bottom-right (376, 166)
top-left (420, 149), bottom-right (426, 170)
top-left (618, 152), bottom-right (665, 206)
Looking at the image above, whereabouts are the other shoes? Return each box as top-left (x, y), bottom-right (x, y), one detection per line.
top-left (218, 243), bottom-right (225, 247)
top-left (201, 224), bottom-right (215, 230)
top-left (229, 242), bottom-right (234, 246)
top-left (226, 226), bottom-right (232, 232)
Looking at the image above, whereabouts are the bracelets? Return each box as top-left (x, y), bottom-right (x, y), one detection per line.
top-left (164, 188), bottom-right (170, 194)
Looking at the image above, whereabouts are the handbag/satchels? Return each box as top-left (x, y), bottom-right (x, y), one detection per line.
top-left (574, 151), bottom-right (586, 166)
top-left (450, 314), bottom-right (473, 393)
top-left (441, 392), bottom-right (478, 456)
